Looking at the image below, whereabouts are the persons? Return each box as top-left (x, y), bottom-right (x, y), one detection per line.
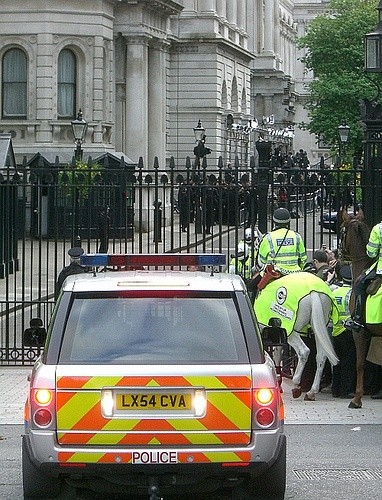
top-left (228, 207), bottom-right (382, 400)
top-left (177, 179), bottom-right (252, 234)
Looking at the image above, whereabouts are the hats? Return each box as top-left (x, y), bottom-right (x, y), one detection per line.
top-left (68, 247), bottom-right (84, 258)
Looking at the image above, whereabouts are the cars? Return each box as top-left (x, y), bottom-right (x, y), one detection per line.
top-left (21, 254), bottom-right (287, 500)
top-left (319, 206), bottom-right (358, 232)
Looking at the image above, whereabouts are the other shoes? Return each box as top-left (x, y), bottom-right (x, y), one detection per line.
top-left (332, 394), bottom-right (339, 397)
top-left (371, 392), bottom-right (382, 399)
top-left (342, 394), bottom-right (353, 398)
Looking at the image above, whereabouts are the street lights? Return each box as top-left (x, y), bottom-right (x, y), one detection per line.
top-left (193, 118), bottom-right (205, 235)
top-left (339, 118), bottom-right (351, 223)
top-left (71, 107), bottom-right (88, 248)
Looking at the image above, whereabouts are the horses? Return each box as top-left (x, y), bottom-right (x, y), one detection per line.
top-left (338, 205), bottom-right (382, 408)
top-left (253, 271), bottom-right (339, 401)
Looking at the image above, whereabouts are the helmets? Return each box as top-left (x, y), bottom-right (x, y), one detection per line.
top-left (273, 207), bottom-right (291, 223)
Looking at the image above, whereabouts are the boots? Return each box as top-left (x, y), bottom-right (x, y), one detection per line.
top-left (345, 294), bottom-right (365, 333)
